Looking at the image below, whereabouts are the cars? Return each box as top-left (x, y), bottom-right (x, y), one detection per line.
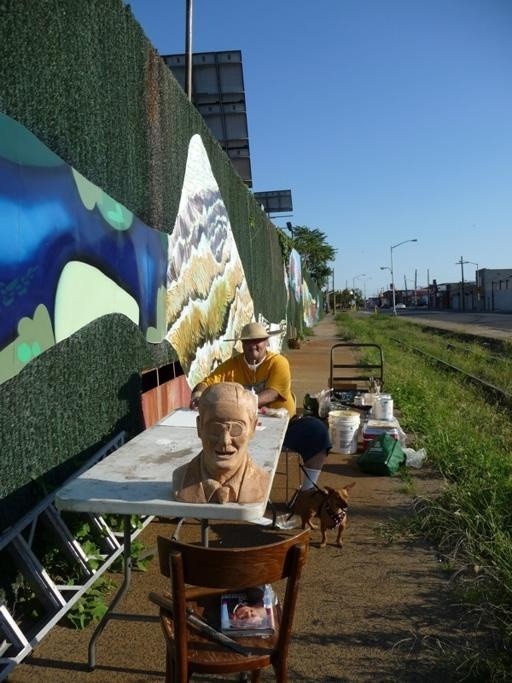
top-left (394, 303), bottom-right (406, 311)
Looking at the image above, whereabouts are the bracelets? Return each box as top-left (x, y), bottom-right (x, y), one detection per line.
top-left (191, 391), bottom-right (203, 400)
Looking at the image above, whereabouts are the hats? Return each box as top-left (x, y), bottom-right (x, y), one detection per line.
top-left (224, 323), bottom-right (285, 341)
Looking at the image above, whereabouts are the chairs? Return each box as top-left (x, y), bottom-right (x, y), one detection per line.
top-left (156, 527), bottom-right (315, 681)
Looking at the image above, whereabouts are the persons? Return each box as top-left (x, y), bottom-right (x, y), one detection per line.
top-left (173, 383), bottom-right (271, 504)
top-left (190, 323), bottom-right (332, 493)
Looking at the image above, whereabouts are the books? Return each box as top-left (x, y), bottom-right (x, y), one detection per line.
top-left (220, 584), bottom-right (275, 638)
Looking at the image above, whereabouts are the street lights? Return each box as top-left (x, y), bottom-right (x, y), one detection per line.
top-left (457, 261), bottom-right (481, 313)
top-left (352, 273), bottom-right (366, 312)
top-left (380, 239), bottom-right (417, 317)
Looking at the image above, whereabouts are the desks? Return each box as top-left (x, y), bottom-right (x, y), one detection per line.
top-left (53, 408), bottom-right (292, 673)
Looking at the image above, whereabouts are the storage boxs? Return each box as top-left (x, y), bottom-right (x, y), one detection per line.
top-left (362, 422), bottom-right (399, 454)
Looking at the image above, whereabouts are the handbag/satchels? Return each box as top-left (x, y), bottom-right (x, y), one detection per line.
top-left (359, 433), bottom-right (407, 475)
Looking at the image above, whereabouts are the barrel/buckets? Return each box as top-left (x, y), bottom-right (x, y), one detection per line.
top-left (374, 399), bottom-right (394, 421)
top-left (328, 410), bottom-right (360, 455)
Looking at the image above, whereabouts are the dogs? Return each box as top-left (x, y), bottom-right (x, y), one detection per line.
top-left (285, 481), bottom-right (357, 548)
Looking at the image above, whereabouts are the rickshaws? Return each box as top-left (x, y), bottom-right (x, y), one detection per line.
top-left (329, 343), bottom-right (384, 412)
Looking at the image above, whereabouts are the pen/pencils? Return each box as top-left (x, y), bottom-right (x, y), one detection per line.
top-left (370, 376), bottom-right (380, 387)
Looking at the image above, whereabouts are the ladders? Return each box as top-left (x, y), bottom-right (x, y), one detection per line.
top-left (0, 430), bottom-right (157, 682)
